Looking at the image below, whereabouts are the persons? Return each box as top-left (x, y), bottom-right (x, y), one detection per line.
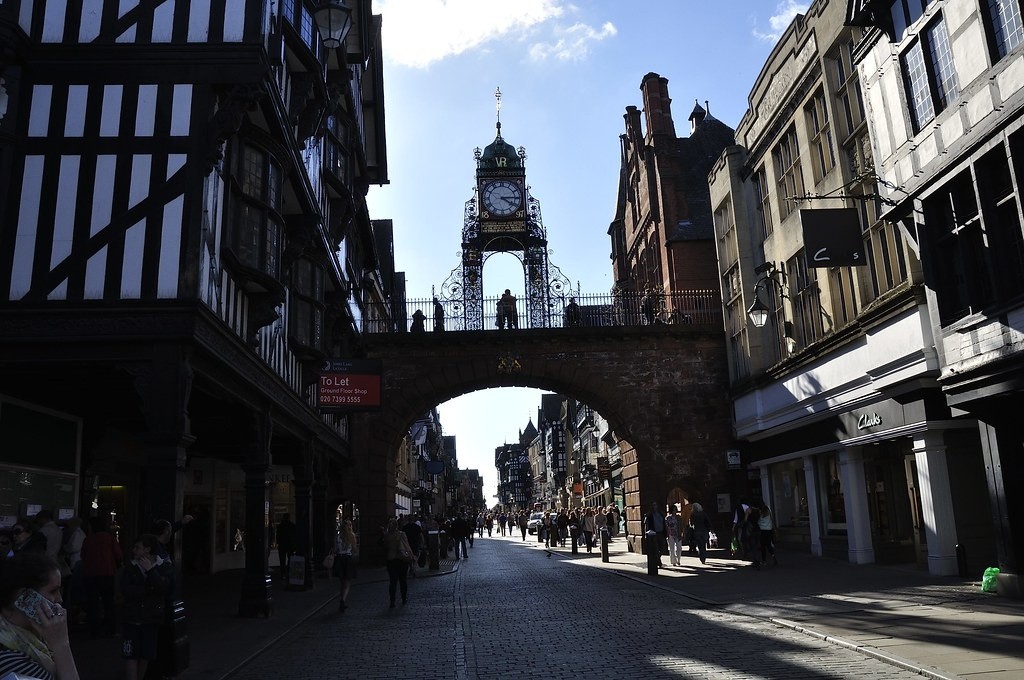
top-left (409, 290), bottom-right (520, 333)
top-left (0, 508), bottom-right (777, 680)
top-left (564, 290), bottom-right (691, 324)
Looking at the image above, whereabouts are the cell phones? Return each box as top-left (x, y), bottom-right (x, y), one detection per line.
top-left (143, 548), bottom-right (150, 558)
top-left (13, 587), bottom-right (60, 627)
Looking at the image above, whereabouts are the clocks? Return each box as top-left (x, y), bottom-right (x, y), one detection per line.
top-left (483, 181), bottom-right (522, 217)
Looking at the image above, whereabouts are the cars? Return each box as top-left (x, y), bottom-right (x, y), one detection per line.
top-left (535, 513), bottom-right (568, 538)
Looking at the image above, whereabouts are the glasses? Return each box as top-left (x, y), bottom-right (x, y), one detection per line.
top-left (12, 527), bottom-right (26, 534)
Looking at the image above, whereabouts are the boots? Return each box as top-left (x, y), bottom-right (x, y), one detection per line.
top-left (389, 593), bottom-right (396, 608)
top-left (402, 592), bottom-right (408, 604)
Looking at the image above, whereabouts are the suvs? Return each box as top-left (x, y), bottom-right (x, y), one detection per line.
top-left (527, 511), bottom-right (546, 535)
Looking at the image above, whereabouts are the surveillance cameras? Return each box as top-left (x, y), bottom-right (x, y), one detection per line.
top-left (754, 262), bottom-right (773, 276)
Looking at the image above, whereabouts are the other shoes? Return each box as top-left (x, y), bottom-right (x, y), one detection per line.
top-left (340, 600), bottom-right (347, 611)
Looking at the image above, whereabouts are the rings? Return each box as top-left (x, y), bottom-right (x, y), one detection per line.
top-left (50, 614), bottom-right (55, 618)
top-left (58, 613), bottom-right (63, 616)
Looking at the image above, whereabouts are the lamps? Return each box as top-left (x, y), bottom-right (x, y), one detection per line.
top-left (746, 276), bottom-right (792, 338)
top-left (268, 0), bottom-right (355, 67)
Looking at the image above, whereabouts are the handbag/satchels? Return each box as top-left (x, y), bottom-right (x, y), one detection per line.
top-left (321, 549), bottom-right (335, 568)
top-left (772, 527), bottom-right (780, 544)
top-left (400, 549), bottom-right (411, 563)
